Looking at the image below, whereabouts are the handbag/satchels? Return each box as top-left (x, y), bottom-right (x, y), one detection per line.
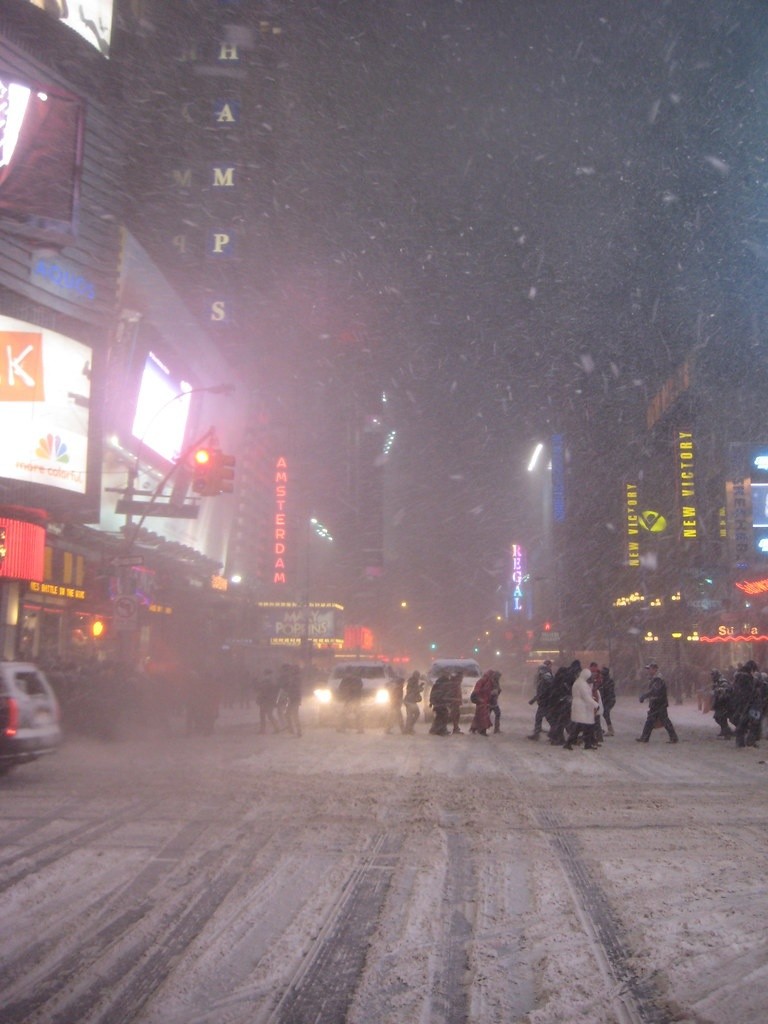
top-left (471, 691), bottom-right (479, 703)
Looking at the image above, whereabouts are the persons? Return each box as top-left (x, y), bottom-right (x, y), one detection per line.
top-left (338, 666), bottom-right (365, 735)
top-left (468, 670), bottom-right (502, 737)
top-left (43, 656), bottom-right (250, 738)
top-left (527, 660), bottom-right (616, 742)
top-left (671, 659), bottom-right (768, 749)
top-left (426, 670), bottom-right (464, 737)
top-left (562, 668), bottom-right (598, 751)
top-left (403, 670), bottom-right (424, 736)
top-left (634, 662), bottom-right (679, 744)
top-left (383, 666), bottom-right (405, 734)
top-left (253, 664), bottom-right (303, 738)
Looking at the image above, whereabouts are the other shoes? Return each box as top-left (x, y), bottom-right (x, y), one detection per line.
top-left (527, 728), bottom-right (604, 750)
top-left (636, 737), bottom-right (649, 743)
top-left (666, 739), bottom-right (678, 743)
top-left (338, 728), bottom-right (503, 736)
top-left (257, 731), bottom-right (304, 739)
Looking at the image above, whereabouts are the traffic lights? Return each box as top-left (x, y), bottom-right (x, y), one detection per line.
top-left (192, 448), bottom-right (215, 495)
top-left (215, 451), bottom-right (235, 493)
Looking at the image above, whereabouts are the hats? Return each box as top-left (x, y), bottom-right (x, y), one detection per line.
top-left (644, 662), bottom-right (658, 669)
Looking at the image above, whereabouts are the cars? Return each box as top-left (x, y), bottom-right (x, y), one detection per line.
top-left (419, 660), bottom-right (484, 723)
top-left (318, 661), bottom-right (405, 727)
top-left (0, 661), bottom-right (62, 776)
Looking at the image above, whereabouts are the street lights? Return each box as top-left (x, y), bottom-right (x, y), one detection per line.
top-left (115, 382), bottom-right (236, 660)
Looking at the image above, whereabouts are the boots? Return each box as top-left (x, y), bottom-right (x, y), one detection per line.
top-left (605, 723), bottom-right (614, 737)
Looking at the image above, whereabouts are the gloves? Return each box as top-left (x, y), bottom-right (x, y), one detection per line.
top-left (640, 696), bottom-right (645, 704)
